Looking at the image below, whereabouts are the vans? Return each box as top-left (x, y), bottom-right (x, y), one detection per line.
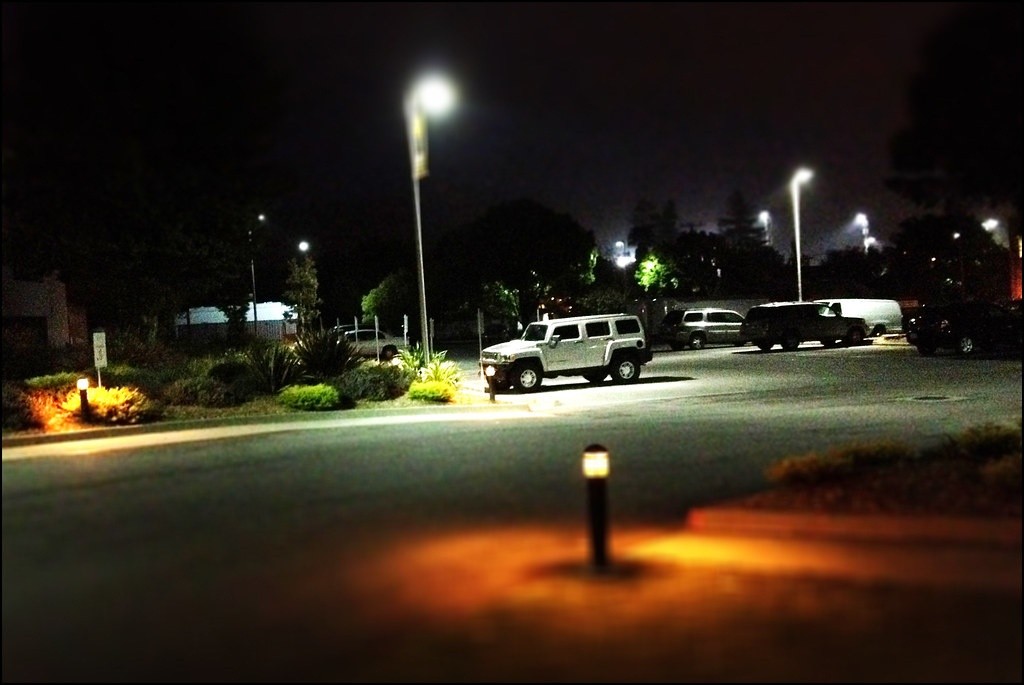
top-left (815, 298), bottom-right (902, 338)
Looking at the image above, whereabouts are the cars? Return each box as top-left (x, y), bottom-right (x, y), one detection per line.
top-left (906, 300), bottom-right (1024, 354)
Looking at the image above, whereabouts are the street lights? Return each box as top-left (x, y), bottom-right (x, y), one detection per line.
top-left (789, 168), bottom-right (816, 301)
top-left (404, 74), bottom-right (458, 370)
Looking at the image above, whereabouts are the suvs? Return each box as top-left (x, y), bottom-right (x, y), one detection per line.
top-left (740, 301), bottom-right (868, 352)
top-left (660, 307), bottom-right (750, 350)
top-left (342, 329), bottom-right (411, 361)
top-left (478, 311), bottom-right (653, 395)
